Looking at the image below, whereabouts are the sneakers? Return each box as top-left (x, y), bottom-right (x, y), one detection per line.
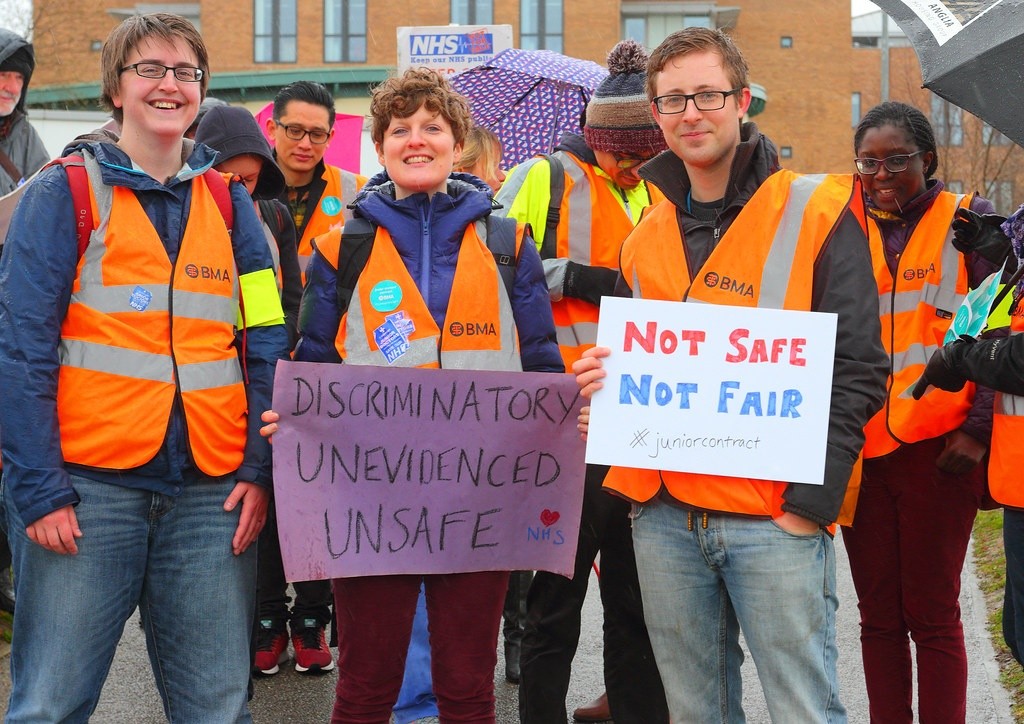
top-left (253, 617), bottom-right (290, 676)
top-left (289, 617), bottom-right (335, 673)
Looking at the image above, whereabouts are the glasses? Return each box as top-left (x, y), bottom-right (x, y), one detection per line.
top-left (610, 150), bottom-right (655, 169)
top-left (854, 149), bottom-right (926, 175)
top-left (118, 63), bottom-right (205, 82)
top-left (653, 86), bottom-right (741, 114)
top-left (275, 118), bottom-right (330, 144)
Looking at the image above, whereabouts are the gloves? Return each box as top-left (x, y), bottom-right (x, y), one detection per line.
top-left (951, 207), bottom-right (1018, 275)
top-left (911, 339), bottom-right (968, 400)
top-left (936, 430), bottom-right (986, 479)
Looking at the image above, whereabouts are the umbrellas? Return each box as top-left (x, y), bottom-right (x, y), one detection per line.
top-left (871, 0), bottom-right (1024, 148)
top-left (443, 47), bottom-right (610, 171)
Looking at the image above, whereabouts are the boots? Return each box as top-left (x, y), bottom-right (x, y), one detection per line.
top-left (502, 571), bottom-right (534, 681)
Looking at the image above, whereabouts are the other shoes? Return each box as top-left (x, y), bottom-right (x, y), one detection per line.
top-left (0, 568), bottom-right (15, 613)
top-left (573, 692), bottom-right (611, 722)
top-left (408, 717), bottom-right (439, 724)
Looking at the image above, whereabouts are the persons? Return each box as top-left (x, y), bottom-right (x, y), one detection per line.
top-left (195, 105), bottom-right (302, 350)
top-left (572, 26), bottom-right (892, 724)
top-left (259, 67), bottom-right (590, 724)
top-left (0, 13), bottom-right (292, 724)
top-left (492, 39), bottom-right (670, 724)
top-left (255, 80), bottom-right (366, 675)
top-left (0, 27), bottom-right (51, 199)
top-left (913, 208), bottom-right (1024, 666)
top-left (841, 102), bottom-right (1001, 724)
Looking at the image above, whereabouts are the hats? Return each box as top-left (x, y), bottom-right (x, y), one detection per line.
top-left (583, 41), bottom-right (668, 152)
top-left (1, 47), bottom-right (34, 78)
